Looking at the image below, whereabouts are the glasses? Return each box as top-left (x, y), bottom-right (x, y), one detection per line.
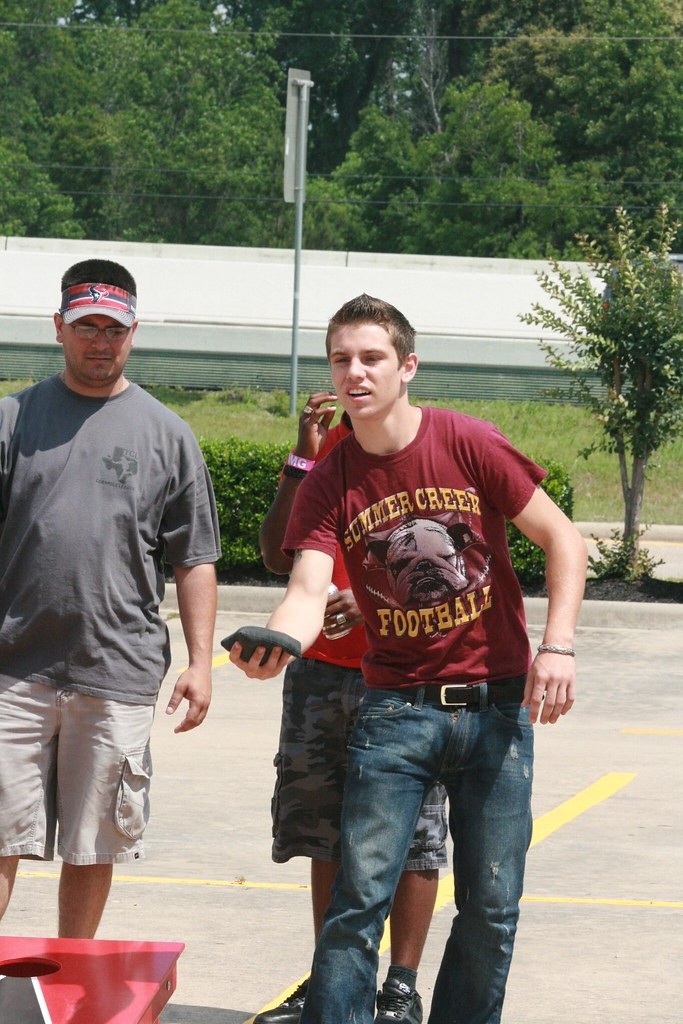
top-left (68, 323), bottom-right (129, 341)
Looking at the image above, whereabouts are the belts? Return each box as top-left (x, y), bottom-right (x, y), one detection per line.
top-left (389, 673), bottom-right (526, 708)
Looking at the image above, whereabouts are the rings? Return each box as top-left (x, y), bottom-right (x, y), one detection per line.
top-left (303, 406), bottom-right (313, 414)
top-left (337, 614), bottom-right (345, 624)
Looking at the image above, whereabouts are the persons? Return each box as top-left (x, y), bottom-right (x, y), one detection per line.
top-left (2, 262), bottom-right (219, 937)
top-left (229, 293), bottom-right (589, 1024)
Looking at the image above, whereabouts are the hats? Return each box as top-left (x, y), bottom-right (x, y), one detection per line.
top-left (59, 282), bottom-right (136, 328)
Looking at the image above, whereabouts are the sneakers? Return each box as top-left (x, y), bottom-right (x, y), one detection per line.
top-left (254, 979), bottom-right (311, 1024)
top-left (374, 978), bottom-right (423, 1024)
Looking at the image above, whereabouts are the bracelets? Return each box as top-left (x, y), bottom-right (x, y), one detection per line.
top-left (537, 645), bottom-right (575, 656)
top-left (283, 454), bottom-right (315, 479)
